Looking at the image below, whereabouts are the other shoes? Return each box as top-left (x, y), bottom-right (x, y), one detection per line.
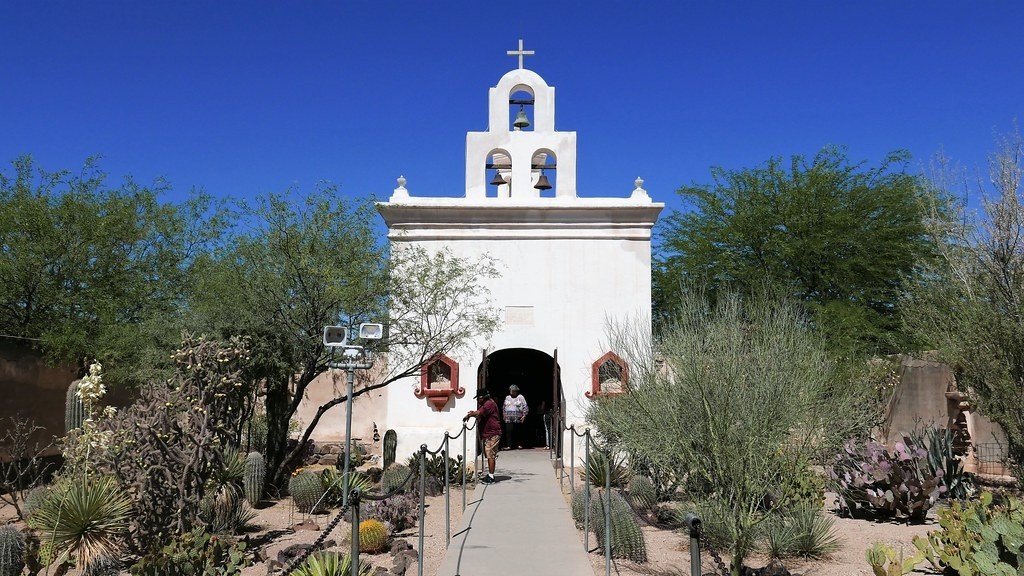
top-left (504, 447), bottom-right (511, 450)
top-left (518, 446), bottom-right (523, 449)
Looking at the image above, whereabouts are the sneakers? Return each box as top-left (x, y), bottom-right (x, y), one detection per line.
top-left (481, 474), bottom-right (495, 484)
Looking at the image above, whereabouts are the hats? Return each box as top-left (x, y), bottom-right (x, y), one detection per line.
top-left (473, 388), bottom-right (489, 399)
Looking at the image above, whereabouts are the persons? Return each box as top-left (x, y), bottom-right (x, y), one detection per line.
top-left (463, 388), bottom-right (502, 485)
top-left (503, 384), bottom-right (529, 450)
